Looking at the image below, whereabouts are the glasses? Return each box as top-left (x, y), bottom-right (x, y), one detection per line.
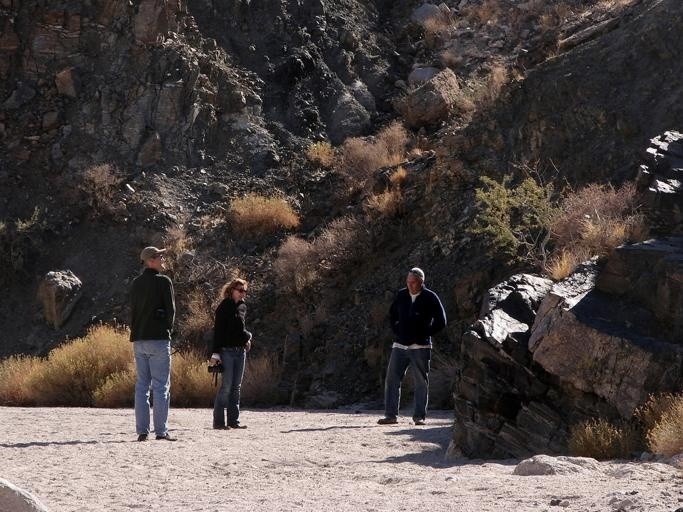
top-left (233, 287), bottom-right (248, 294)
top-left (409, 269), bottom-right (423, 278)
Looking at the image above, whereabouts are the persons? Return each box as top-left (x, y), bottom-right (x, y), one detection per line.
top-left (377, 267), bottom-right (448, 427)
top-left (208, 277), bottom-right (253, 429)
top-left (125, 246), bottom-right (176, 441)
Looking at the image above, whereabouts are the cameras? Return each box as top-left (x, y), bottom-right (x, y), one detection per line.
top-left (208, 364), bottom-right (225, 373)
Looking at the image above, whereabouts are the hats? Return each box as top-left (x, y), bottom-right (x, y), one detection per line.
top-left (140, 246), bottom-right (167, 264)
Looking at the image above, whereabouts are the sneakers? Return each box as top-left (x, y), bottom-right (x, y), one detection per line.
top-left (412, 417), bottom-right (427, 425)
top-left (214, 425), bottom-right (231, 431)
top-left (228, 424), bottom-right (248, 429)
top-left (377, 417), bottom-right (397, 424)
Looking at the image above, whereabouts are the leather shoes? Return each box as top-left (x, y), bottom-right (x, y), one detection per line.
top-left (137, 434), bottom-right (149, 443)
top-left (155, 433), bottom-right (178, 441)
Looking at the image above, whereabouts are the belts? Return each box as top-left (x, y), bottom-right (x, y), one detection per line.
top-left (221, 345), bottom-right (246, 352)
